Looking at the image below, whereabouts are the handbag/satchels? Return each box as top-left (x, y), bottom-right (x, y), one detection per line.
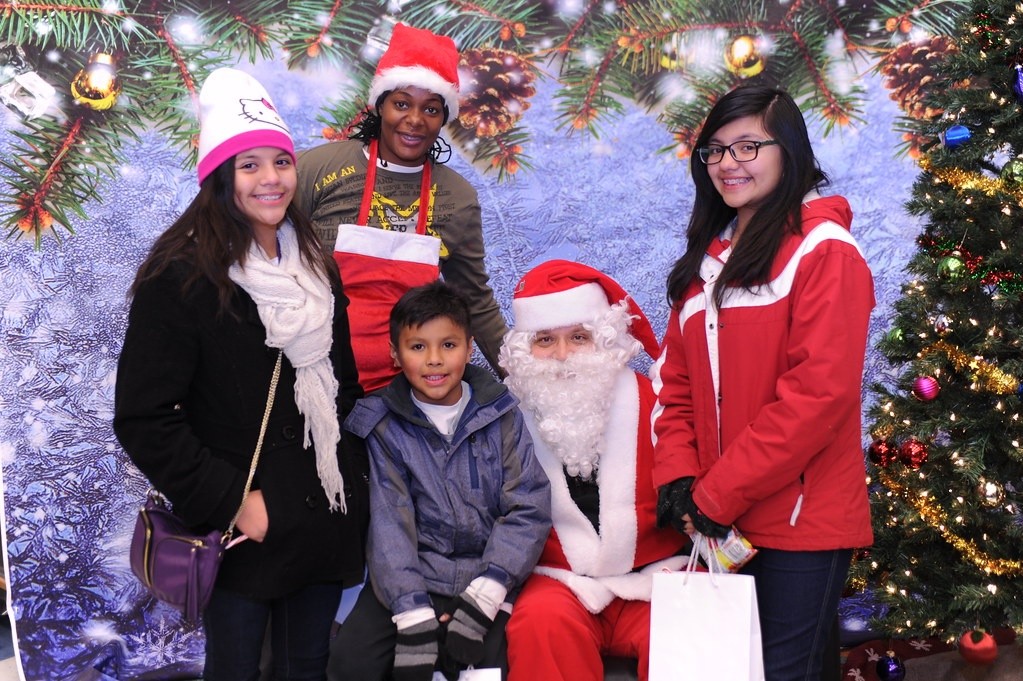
top-left (130, 493), bottom-right (223, 622)
top-left (648, 530), bottom-right (766, 681)
top-left (431, 664), bottom-right (502, 681)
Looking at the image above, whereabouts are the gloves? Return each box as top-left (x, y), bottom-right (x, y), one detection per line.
top-left (393, 617), bottom-right (447, 681)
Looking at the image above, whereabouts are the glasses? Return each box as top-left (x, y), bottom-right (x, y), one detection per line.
top-left (696, 140), bottom-right (780, 165)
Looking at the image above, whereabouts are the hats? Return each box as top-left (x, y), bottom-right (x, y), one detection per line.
top-left (368, 22), bottom-right (460, 125)
top-left (196, 67), bottom-right (296, 188)
top-left (512, 259), bottom-right (660, 381)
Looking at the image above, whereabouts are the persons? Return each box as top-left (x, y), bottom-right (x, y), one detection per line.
top-left (649, 86), bottom-right (876, 681)
top-left (113, 66), bottom-right (366, 681)
top-left (294, 22), bottom-right (511, 396)
top-left (499, 260), bottom-right (709, 681)
top-left (344, 280), bottom-right (552, 681)
